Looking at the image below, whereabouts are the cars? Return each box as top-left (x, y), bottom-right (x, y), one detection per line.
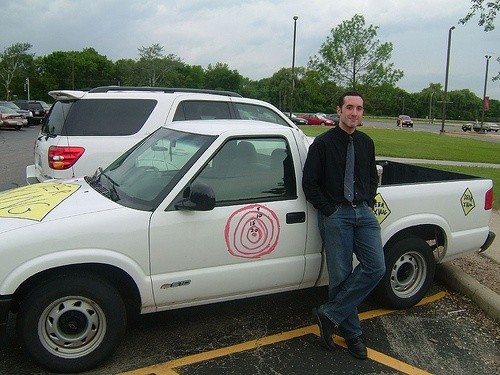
top-left (462, 121), bottom-right (499, 133)
top-left (282, 112), bottom-right (363, 126)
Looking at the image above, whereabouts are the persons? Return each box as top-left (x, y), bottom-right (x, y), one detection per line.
top-left (302, 91), bottom-right (386, 357)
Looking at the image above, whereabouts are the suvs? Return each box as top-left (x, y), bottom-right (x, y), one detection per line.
top-left (0, 100), bottom-right (52, 131)
top-left (397, 115), bottom-right (414, 127)
top-left (26, 85), bottom-right (316, 186)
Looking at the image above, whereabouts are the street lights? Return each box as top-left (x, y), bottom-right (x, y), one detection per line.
top-left (440, 26), bottom-right (456, 134)
top-left (479, 55), bottom-right (492, 134)
top-left (288, 16), bottom-right (298, 119)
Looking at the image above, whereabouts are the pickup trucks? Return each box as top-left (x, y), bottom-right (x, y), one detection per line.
top-left (0, 120), bottom-right (495, 375)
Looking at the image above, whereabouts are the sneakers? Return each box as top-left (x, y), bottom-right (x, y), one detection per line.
top-left (348, 335), bottom-right (367, 359)
top-left (316, 310), bottom-right (337, 351)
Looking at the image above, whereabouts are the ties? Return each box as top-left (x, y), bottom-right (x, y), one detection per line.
top-left (344, 136), bottom-right (354, 202)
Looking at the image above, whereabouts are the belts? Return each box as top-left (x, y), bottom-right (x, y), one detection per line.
top-left (343, 202), bottom-right (362, 207)
